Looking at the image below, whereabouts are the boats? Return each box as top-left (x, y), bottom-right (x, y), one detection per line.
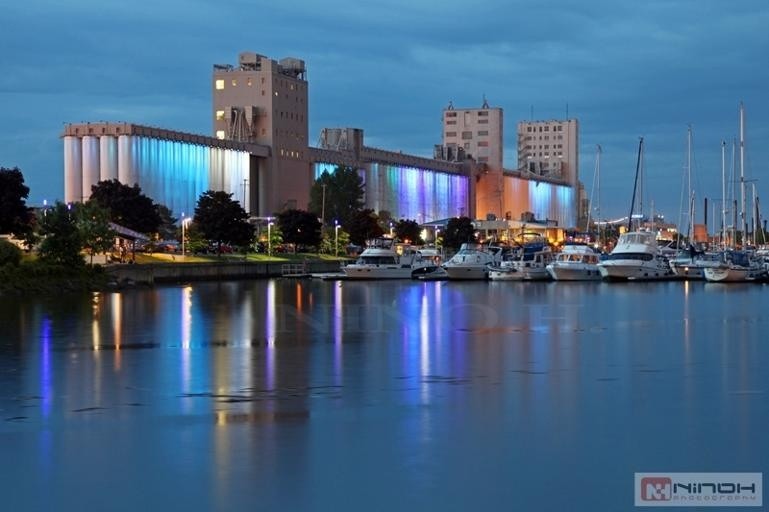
top-left (441, 236), bottom-right (503, 281)
top-left (340, 233), bottom-right (441, 279)
top-left (524, 264), bottom-right (548, 280)
top-left (544, 244), bottom-right (602, 281)
top-left (487, 244), bottom-right (554, 280)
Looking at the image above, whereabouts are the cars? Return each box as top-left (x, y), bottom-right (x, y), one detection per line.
top-left (128, 238), bottom-right (317, 257)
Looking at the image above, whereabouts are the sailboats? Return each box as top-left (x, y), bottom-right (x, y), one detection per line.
top-left (584, 102), bottom-right (769, 283)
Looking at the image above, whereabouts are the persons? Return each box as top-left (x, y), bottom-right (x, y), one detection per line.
top-left (126, 241), bottom-right (131, 254)
top-left (119, 243), bottom-right (126, 263)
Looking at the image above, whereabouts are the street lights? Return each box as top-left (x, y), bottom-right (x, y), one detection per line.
top-left (434, 226), bottom-right (439, 249)
top-left (180, 212), bottom-right (184, 256)
top-left (267, 217), bottom-right (274, 256)
top-left (389, 222), bottom-right (395, 236)
top-left (334, 221), bottom-right (341, 257)
